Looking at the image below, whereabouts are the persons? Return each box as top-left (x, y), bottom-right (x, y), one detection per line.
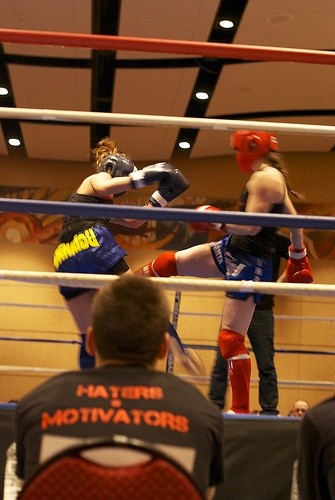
top-left (14, 273), bottom-right (225, 500)
top-left (297, 398), bottom-right (335, 500)
top-left (133, 129), bottom-right (313, 414)
top-left (292, 400), bottom-right (310, 417)
top-left (209, 234), bottom-right (291, 413)
top-left (52, 137), bottom-right (203, 374)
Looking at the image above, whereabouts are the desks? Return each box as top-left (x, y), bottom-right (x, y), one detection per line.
top-left (0, 402), bottom-right (309, 500)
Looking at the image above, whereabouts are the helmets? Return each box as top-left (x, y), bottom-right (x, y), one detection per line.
top-left (231, 129), bottom-right (278, 174)
top-left (96, 155), bottom-right (133, 198)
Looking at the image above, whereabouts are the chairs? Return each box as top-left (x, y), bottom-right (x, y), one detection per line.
top-left (14, 440), bottom-right (207, 500)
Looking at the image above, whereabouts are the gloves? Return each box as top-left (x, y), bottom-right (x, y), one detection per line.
top-left (287, 244), bottom-right (314, 283)
top-left (189, 205), bottom-right (224, 234)
top-left (129, 162), bottom-right (175, 189)
top-left (149, 169), bottom-right (191, 208)
